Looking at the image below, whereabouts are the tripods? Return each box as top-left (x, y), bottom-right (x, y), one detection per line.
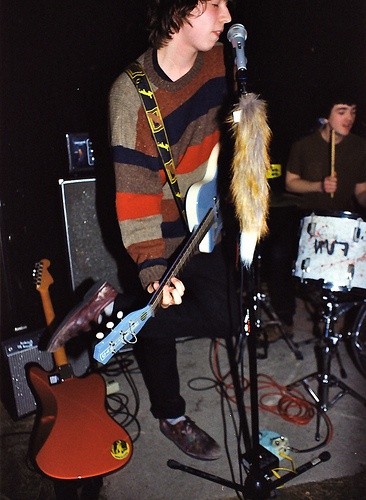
top-left (166, 73), bottom-right (366, 500)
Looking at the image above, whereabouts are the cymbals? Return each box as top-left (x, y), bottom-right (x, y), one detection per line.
top-left (270, 188), bottom-right (306, 208)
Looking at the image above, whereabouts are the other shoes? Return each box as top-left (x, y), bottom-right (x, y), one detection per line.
top-left (159, 417), bottom-right (222, 460)
top-left (320, 313), bottom-right (344, 338)
top-left (259, 321), bottom-right (293, 346)
top-left (47, 279), bottom-right (117, 355)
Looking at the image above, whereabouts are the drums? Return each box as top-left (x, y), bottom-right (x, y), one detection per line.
top-left (293, 214), bottom-right (366, 293)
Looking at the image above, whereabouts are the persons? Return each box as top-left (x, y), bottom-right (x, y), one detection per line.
top-left (260, 90), bottom-right (366, 350)
top-left (47, 0), bottom-right (248, 461)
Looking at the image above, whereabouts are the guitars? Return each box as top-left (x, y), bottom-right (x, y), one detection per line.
top-left (24, 258), bottom-right (134, 481)
top-left (92, 142), bottom-right (224, 366)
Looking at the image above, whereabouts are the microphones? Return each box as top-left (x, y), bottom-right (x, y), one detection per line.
top-left (227, 24), bottom-right (247, 76)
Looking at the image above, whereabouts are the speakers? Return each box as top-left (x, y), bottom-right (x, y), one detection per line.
top-left (0, 323), bottom-right (54, 419)
top-left (55, 177), bottom-right (154, 324)
